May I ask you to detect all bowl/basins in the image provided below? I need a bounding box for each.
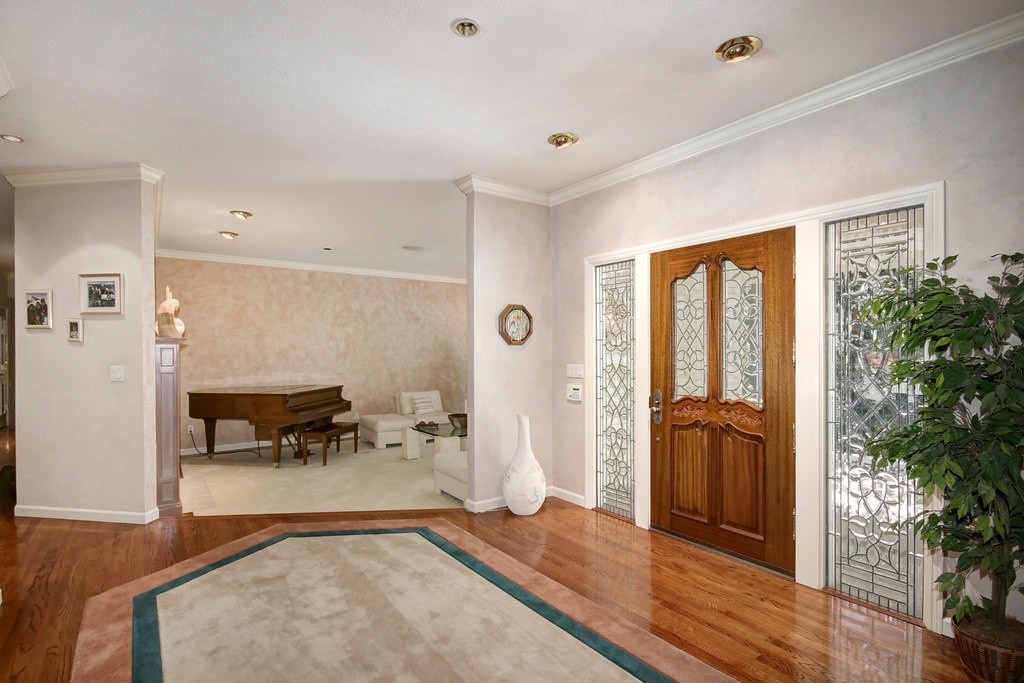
[448,413,467,429]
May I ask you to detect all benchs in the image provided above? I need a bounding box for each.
[302,420,359,466]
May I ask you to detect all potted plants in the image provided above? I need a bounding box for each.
[860,252,1022,683]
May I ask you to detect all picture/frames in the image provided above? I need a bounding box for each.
[79,272,125,315]
[68,319,85,343]
[23,289,54,330]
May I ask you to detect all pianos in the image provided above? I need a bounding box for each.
[185,383,352,468]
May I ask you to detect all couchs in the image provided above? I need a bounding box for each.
[361,389,458,450]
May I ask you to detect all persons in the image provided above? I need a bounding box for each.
[69,322,78,339]
[28,298,48,325]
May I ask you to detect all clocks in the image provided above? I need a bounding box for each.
[497,303,534,345]
